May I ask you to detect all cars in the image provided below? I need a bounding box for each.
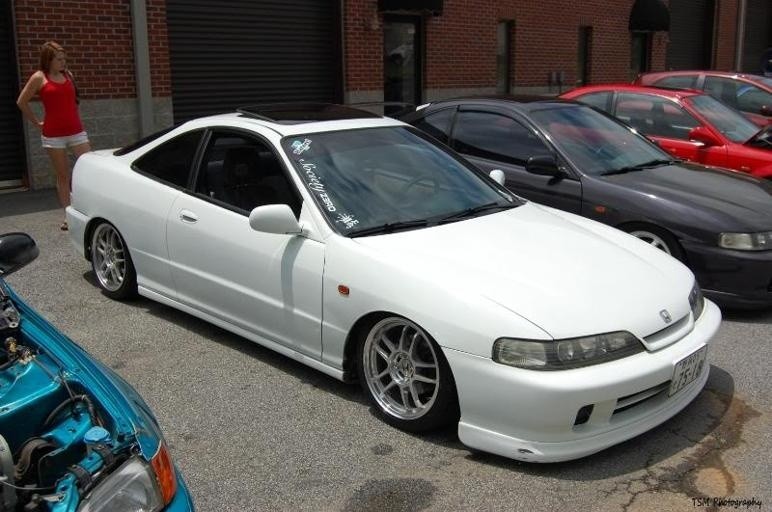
[2,227,197,510]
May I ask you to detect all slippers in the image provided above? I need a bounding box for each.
[61,221,67,229]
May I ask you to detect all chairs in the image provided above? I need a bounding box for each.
[630,103,688,140]
[216,144,282,212]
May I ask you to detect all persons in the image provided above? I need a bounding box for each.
[16,41,92,230]
